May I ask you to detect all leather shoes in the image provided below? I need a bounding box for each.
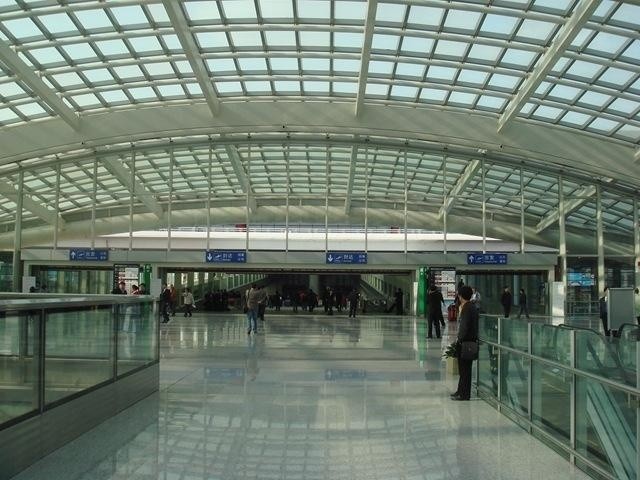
[450,392,470,400]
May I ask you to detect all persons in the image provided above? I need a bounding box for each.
[450,284,479,401]
[438,292,445,329]
[599,286,609,336]
[29,287,38,321]
[634,287,640,325]
[425,286,441,339]
[110,282,361,335]
[501,287,512,318]
[516,289,529,319]
[469,287,482,311]
[394,288,403,315]
[40,285,49,321]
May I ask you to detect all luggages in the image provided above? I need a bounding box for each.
[448,303,457,321]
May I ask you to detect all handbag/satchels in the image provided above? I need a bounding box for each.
[243,305,247,313]
[460,341,479,360]
